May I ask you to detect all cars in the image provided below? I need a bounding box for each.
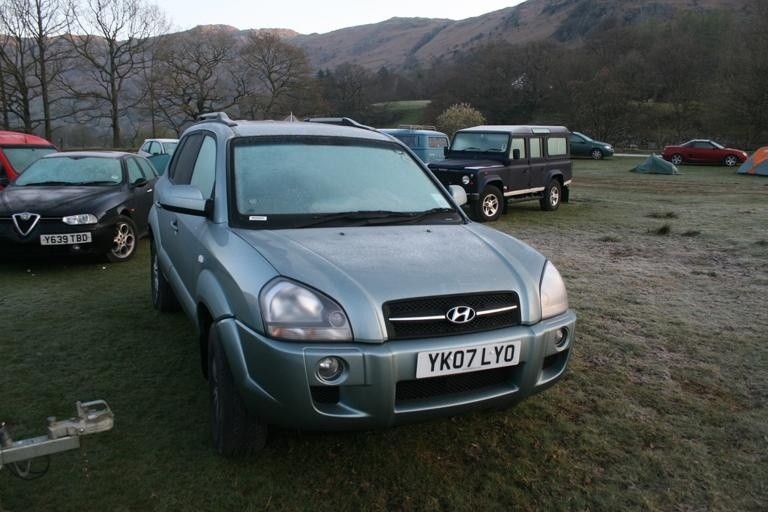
[0,129,181,261]
[661,140,748,167]
[570,132,614,160]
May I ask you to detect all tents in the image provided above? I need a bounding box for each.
[629,153,678,175]
[735,146,768,177]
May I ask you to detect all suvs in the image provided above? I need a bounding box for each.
[149,111,577,459]
[424,126,572,221]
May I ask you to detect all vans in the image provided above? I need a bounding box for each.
[377,128,450,164]
